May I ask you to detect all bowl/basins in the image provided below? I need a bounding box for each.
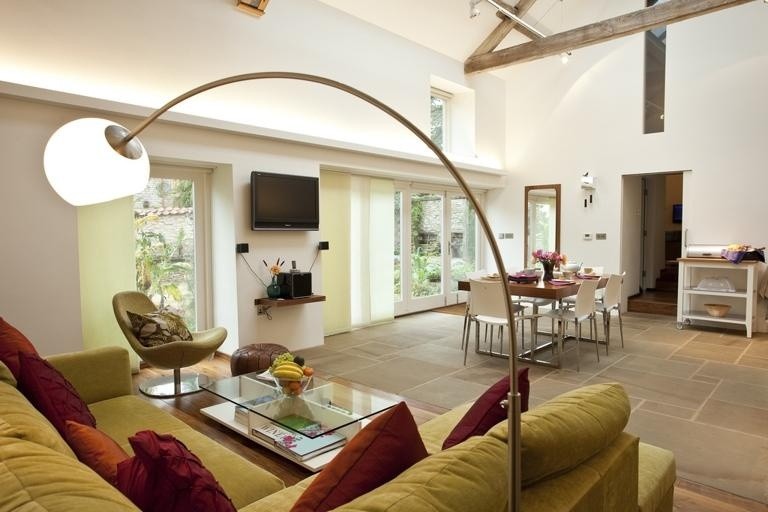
[703,304,731,317]
[274,376,311,398]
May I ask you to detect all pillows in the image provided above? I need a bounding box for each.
[0,318,40,382]
[287,402,426,512]
[115,428,237,512]
[125,304,195,348]
[438,366,529,450]
[15,348,95,434]
[67,418,130,486]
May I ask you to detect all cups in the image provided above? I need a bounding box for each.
[552,272,560,278]
[563,271,571,280]
[536,270,543,277]
[584,268,592,274]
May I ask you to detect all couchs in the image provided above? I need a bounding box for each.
[0,345,285,511]
[234,381,676,512]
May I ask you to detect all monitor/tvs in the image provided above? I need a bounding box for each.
[277,272,313,299]
[251,171,320,232]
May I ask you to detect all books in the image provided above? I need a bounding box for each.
[252,414,317,445]
[256,366,274,381]
[275,424,346,462]
[234,395,275,426]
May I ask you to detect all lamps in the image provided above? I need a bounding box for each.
[42,72,522,511]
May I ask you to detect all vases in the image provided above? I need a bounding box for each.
[542,263,554,281]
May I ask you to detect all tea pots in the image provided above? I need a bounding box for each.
[560,256,584,272]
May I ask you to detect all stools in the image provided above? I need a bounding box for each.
[230,342,291,375]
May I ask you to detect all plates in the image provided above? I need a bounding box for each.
[551,280,574,282]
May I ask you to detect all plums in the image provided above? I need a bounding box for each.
[294,356,304,366]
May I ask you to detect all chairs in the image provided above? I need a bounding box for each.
[461,266,626,373]
[110,291,226,398]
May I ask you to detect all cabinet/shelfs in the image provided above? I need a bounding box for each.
[676,258,758,338]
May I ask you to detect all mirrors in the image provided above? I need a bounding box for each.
[525,184,561,268]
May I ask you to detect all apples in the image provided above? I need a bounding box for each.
[292,387,302,395]
[303,368,313,376]
[288,382,299,390]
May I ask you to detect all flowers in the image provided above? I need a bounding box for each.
[531,249,566,269]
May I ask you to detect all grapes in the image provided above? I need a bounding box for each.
[269,352,294,373]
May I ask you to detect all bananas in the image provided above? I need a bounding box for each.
[272,361,304,379]
